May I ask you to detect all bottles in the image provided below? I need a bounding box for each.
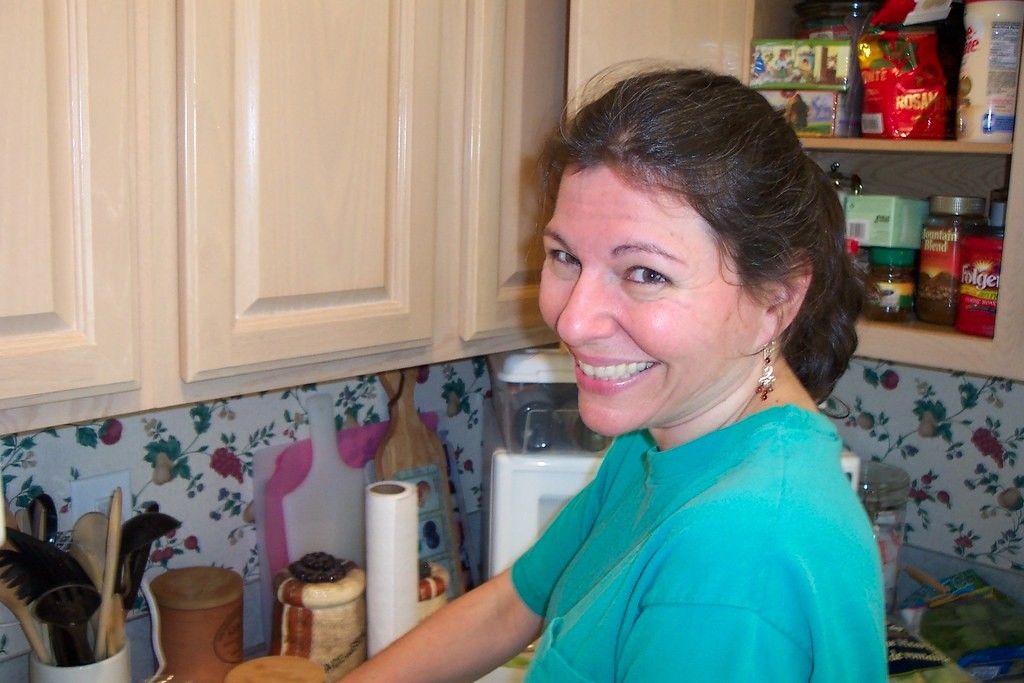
[857,461,910,618]
[955,1,1024,145]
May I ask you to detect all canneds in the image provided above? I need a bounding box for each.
[859,195,1008,339]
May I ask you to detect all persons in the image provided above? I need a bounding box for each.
[339,71,890,683]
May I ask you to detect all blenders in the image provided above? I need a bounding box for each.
[482,341,612,587]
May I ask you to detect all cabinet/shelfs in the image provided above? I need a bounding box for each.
[0,0,1024,435]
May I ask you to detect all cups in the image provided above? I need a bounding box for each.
[30,633,132,683]
[150,565,245,683]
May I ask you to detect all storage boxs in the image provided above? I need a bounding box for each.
[488,343,612,456]
[756,89,858,137]
[845,195,930,248]
[749,40,857,93]
